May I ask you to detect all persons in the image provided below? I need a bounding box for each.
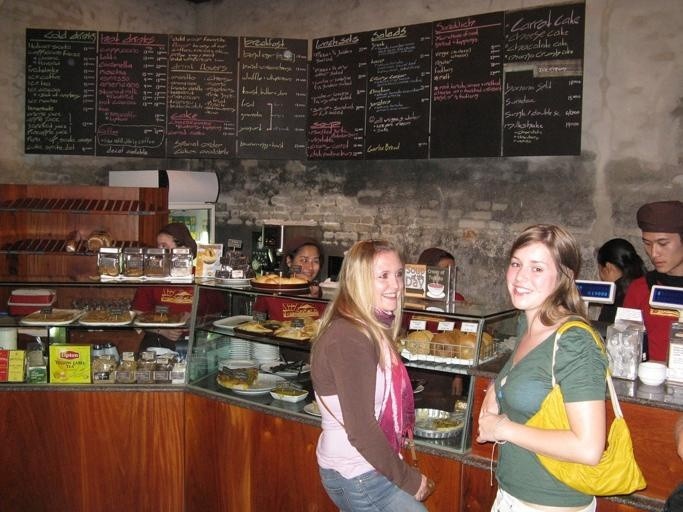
[666,419,682,512]
[475,221,612,512]
[596,237,649,331]
[308,240,437,511]
[621,200,682,362]
[255,236,330,334]
[131,221,198,362]
[401,247,467,398]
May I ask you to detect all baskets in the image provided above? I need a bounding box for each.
[397,339,499,369]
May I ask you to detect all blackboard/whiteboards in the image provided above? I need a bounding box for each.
[307,2,586,160]
[24,27,307,159]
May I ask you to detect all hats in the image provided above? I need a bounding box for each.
[417,247,446,269]
[284,235,324,269]
[162,219,197,261]
[636,201,682,232]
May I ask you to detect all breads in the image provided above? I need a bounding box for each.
[253,272,308,284]
[235,315,314,341]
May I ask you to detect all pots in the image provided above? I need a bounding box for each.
[87,229,110,251]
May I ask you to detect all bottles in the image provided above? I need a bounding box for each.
[200,221,208,241]
[91,342,185,384]
[664,321,683,386]
[168,214,196,239]
[202,257,216,282]
[97,247,190,278]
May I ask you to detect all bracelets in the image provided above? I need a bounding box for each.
[491,418,508,445]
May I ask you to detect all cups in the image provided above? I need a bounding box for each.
[635,384,673,402]
[74,296,135,310]
[638,361,674,385]
[427,283,444,295]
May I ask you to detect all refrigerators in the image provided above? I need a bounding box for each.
[107,169,220,244]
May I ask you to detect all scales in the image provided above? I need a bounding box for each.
[573,279,616,341]
[649,284,683,337]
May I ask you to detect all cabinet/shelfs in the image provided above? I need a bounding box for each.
[0,278,196,384]
[188,289,512,456]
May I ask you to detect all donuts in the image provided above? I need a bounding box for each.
[406,328,493,359]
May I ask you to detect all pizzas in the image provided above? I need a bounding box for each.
[216,366,259,390]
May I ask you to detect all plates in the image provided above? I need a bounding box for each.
[260,360,312,376]
[213,371,286,398]
[302,403,321,420]
[409,405,461,439]
[211,277,251,286]
[413,384,424,394]
[426,291,445,298]
[132,314,190,326]
[19,308,83,326]
[217,337,281,371]
[211,314,252,330]
[79,311,135,326]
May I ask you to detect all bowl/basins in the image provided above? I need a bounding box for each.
[269,387,308,403]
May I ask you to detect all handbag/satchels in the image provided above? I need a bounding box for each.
[523,383,646,497]
